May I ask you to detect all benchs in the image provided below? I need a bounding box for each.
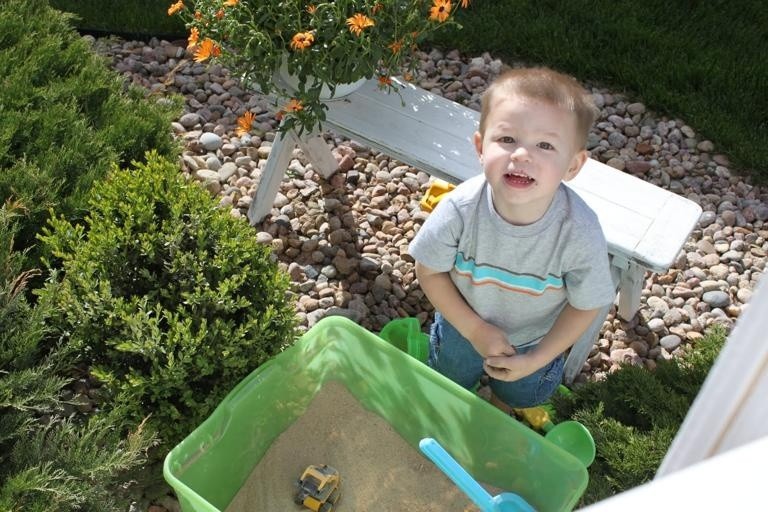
[241,70,703,383]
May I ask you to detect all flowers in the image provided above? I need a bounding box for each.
[168,0,470,138]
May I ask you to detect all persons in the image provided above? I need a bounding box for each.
[408,65,616,406]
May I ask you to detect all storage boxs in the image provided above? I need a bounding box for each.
[163,316,590,511]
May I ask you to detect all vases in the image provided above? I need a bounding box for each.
[278,64,367,100]
